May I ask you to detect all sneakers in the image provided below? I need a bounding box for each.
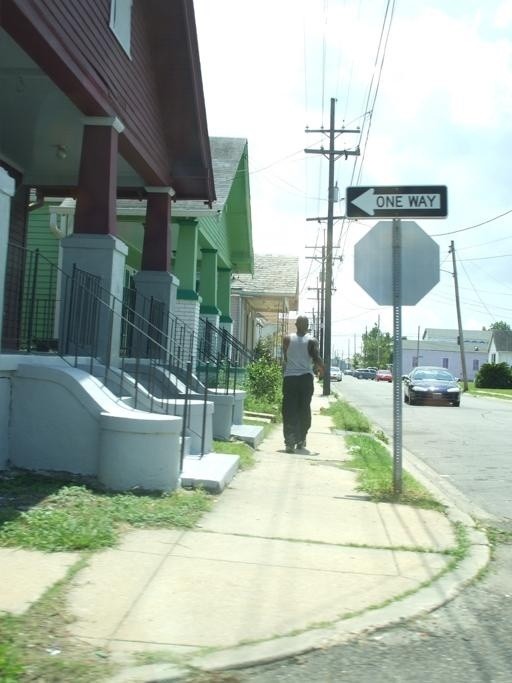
[286,443,295,452]
[297,440,306,448]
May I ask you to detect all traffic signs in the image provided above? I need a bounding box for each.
[346,185,448,219]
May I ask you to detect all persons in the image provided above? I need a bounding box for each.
[279,314,325,455]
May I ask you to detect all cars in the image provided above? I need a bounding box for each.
[344,367,393,382]
[319,366,342,381]
[404,365,461,407]
